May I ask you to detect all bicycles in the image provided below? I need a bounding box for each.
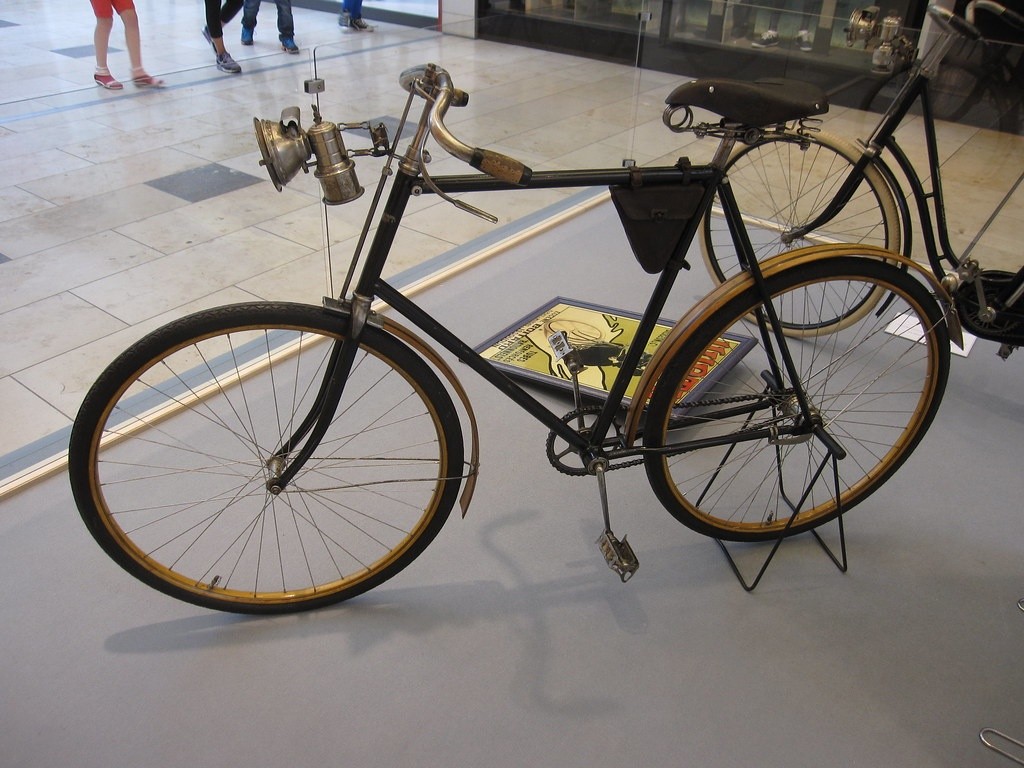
[698,1,1023,362]
[66,49,966,616]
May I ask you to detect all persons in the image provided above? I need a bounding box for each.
[202,0,244,74]
[90,0,166,90]
[340,0,377,35]
[242,1,303,56]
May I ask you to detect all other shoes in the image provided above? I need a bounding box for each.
[795,35,813,52]
[281,38,299,53]
[751,30,780,48]
[240,28,254,45]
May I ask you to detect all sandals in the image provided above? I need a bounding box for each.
[133,76,168,88]
[93,74,123,89]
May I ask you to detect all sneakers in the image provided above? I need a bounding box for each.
[216,52,242,73]
[201,26,219,56]
[338,11,374,33]
